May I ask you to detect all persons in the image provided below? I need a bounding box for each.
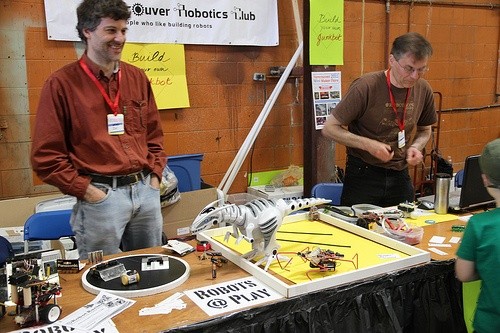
[455,139,500,333]
[322,32,437,208]
[30,0,167,258]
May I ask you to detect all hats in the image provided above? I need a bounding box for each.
[476,138,500,187]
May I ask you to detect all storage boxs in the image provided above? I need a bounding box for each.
[161,153,218,240]
[247,185,303,205]
[0,226,51,250]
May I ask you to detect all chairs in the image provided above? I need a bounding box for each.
[24,210,76,253]
[455,169,464,190]
[312,183,344,206]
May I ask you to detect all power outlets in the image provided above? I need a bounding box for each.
[253,72,266,81]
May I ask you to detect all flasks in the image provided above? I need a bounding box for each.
[434,173,451,214]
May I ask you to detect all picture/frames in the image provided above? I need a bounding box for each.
[197,211,431,298]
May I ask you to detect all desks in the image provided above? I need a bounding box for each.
[0,187,500,333]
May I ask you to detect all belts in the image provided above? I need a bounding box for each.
[79,167,151,186]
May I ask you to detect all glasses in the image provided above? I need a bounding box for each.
[390,53,430,75]
